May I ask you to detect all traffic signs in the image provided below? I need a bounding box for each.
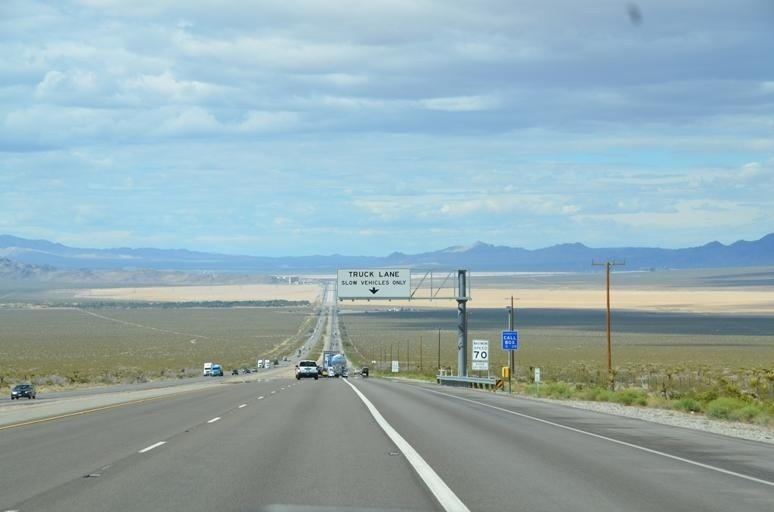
[336,268,412,298]
[501,330,518,350]
[472,340,488,361]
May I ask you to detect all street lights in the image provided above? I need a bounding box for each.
[504,305,513,371]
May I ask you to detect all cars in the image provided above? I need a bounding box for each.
[9,385,34,399]
[201,350,368,381]
